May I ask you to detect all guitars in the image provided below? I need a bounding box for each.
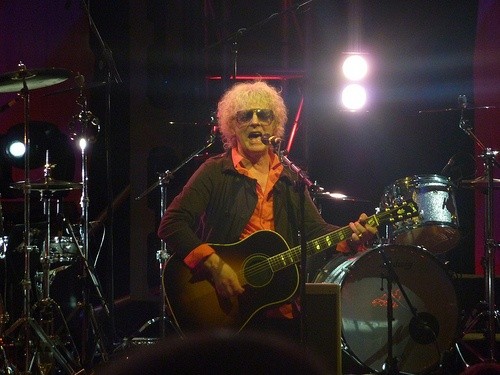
[162,198,419,348]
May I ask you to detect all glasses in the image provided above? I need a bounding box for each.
[236,109,275,125]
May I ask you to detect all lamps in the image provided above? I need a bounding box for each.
[0,121,44,170]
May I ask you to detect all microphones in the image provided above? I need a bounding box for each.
[441,155,455,174]
[458,95,467,111]
[261,134,282,145]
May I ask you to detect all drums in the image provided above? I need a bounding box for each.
[41,236,81,266]
[383,175,460,255]
[314,244,462,374]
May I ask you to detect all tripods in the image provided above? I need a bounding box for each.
[453,106,499,375]
[0,0,221,375]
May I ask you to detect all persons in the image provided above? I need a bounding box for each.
[157,81,380,337]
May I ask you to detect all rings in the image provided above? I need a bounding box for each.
[359,233,363,237]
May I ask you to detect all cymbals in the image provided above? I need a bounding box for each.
[9,181,81,190]
[417,105,496,114]
[458,179,500,189]
[0,68,70,92]
